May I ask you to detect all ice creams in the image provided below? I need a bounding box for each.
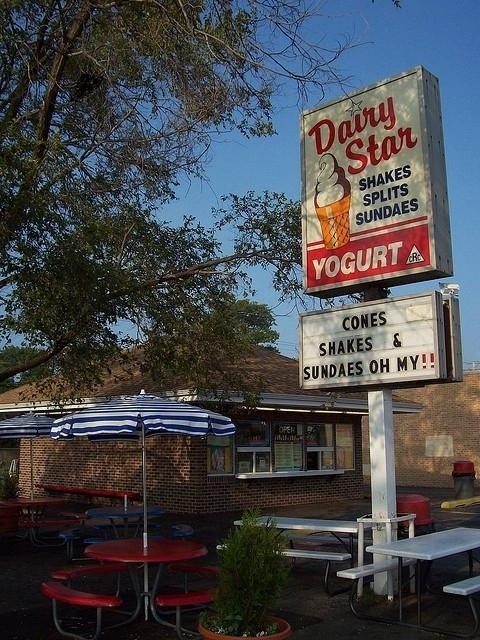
[315,153,352,250]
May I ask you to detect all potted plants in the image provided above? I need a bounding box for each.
[198,507,293,639]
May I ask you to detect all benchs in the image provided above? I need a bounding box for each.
[0,485,164,563]
[40,537,234,640]
[442,575,480,640]
[337,558,418,622]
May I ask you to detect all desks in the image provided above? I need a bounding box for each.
[365,526,479,627]
[215,515,371,596]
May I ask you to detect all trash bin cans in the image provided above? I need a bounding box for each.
[452,461,476,500]
[396,494,436,593]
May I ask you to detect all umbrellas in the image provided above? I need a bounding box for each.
[0,410,59,500]
[50,388,238,621]
